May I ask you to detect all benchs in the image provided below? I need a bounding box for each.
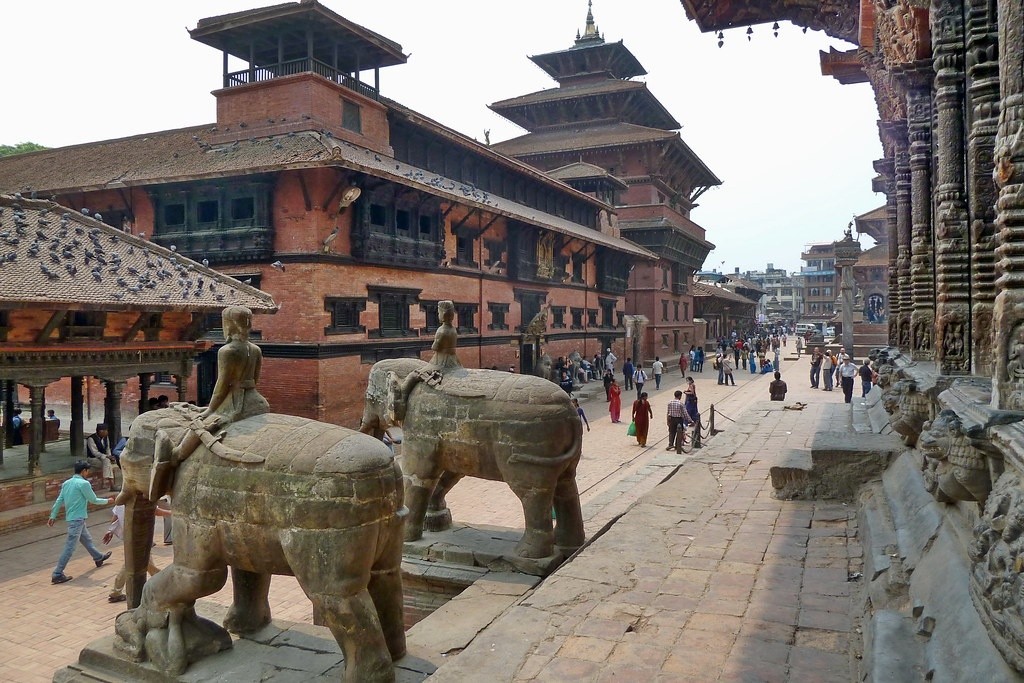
[0,463,116,503]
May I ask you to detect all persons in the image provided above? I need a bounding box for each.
[47,459,114,583]
[677,353,688,378]
[149,394,169,409]
[810,344,878,403]
[769,370,787,401]
[151,494,172,547]
[556,348,617,397]
[632,392,653,447]
[13,407,27,446]
[652,356,664,391]
[690,344,705,373]
[101,500,172,604]
[87,423,122,492]
[48,409,57,419]
[665,376,699,450]
[113,426,131,471]
[572,397,590,432]
[401,299,463,405]
[717,321,809,386]
[867,297,886,325]
[622,357,648,399]
[170,306,270,463]
[603,358,621,423]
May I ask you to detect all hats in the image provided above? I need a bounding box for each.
[843,357,850,359]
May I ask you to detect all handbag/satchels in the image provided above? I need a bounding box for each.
[627,418,636,435]
[716,366,718,370]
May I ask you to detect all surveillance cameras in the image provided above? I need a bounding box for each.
[272,261,281,267]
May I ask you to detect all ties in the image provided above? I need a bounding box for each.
[638,372,639,380]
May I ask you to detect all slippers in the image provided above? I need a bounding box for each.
[164,541,173,545]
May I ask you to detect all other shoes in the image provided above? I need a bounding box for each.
[95,550,112,567]
[52,576,73,583]
[811,385,814,388]
[814,386,818,389]
[110,486,120,492]
[666,446,674,450]
[718,382,724,385]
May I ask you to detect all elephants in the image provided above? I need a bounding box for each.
[114,404,406,683]
[358,357,587,557]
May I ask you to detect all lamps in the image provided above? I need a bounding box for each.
[339,186,361,208]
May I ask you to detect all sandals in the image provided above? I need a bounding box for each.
[108,594,127,602]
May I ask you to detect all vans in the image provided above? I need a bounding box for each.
[795,323,817,336]
[826,326,835,336]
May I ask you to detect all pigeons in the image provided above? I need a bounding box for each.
[171,112,501,211]
[0,191,284,301]
